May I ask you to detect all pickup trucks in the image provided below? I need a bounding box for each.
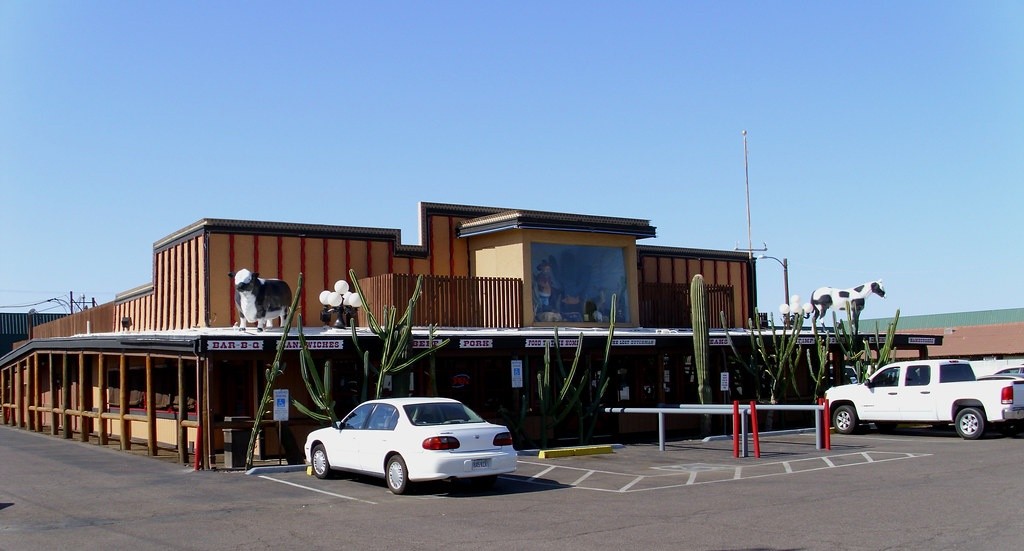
[820,359,1024,440]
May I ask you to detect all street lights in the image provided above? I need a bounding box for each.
[758,254,791,329]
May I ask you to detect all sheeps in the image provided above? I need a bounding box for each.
[227,267,292,333]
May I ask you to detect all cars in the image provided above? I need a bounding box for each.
[993,366,1024,377]
[304,397,518,495]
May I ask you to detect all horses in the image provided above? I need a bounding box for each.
[809,279,887,333]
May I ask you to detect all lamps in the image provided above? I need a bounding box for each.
[320,280,362,331]
[780,295,814,330]
